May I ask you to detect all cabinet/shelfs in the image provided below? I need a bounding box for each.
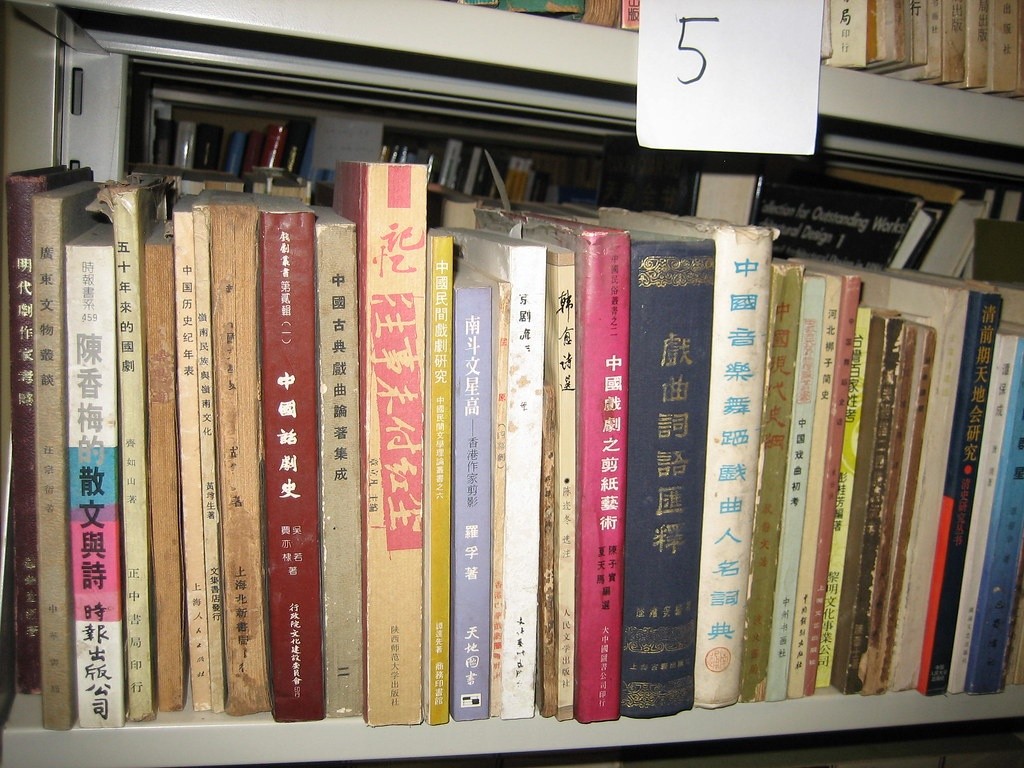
[0,0,1024,768]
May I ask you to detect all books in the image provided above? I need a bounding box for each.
[4,91,1022,726]
[451,0,1024,94]
